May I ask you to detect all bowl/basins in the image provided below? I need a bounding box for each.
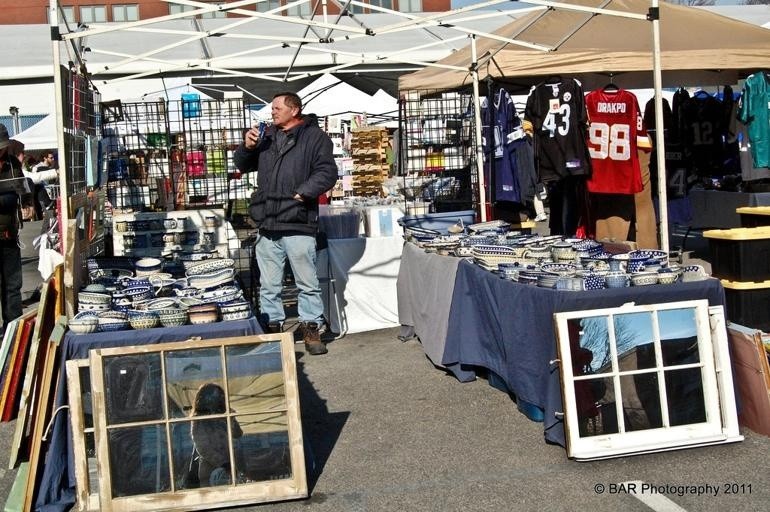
[82,259,238,302]
[67,291,253,336]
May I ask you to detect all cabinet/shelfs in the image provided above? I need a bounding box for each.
[112,209,230,262]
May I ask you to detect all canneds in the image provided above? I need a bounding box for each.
[258,122,267,140]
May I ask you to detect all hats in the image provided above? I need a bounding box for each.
[0,123,11,149]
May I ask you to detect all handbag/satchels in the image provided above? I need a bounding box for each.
[175,445,200,488]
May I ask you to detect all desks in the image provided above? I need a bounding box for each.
[318,200,430,237]
[34,252,266,511]
[397,232,726,445]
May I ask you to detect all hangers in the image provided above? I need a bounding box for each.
[477,58,770,105]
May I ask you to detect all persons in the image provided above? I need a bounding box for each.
[0,122,59,336]
[235,91,339,355]
[75,185,104,240]
[190,382,243,487]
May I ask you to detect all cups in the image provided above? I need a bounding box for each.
[116,216,216,257]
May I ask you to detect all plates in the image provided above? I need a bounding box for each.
[407,219,705,291]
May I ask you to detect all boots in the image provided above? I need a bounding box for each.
[267,321,284,333]
[301,321,327,355]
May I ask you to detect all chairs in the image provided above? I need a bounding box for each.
[241,231,331,328]
